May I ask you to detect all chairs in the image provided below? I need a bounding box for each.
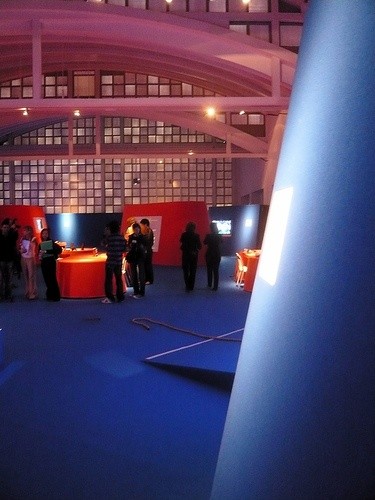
[235,253,248,285]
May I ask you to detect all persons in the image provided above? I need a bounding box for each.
[204,222,224,291]
[31,228,62,302]
[0,218,18,303]
[139,218,155,285]
[178,222,202,293]
[100,221,127,304]
[125,223,149,300]
[16,225,39,301]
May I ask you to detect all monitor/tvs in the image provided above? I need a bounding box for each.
[213,220,232,237]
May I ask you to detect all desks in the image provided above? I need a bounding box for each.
[234,250,259,293]
[56,248,128,297]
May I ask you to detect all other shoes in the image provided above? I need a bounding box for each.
[145,281,153,285]
[102,298,115,303]
[129,294,135,297]
[29,293,37,299]
[133,294,145,299]
[117,295,125,302]
